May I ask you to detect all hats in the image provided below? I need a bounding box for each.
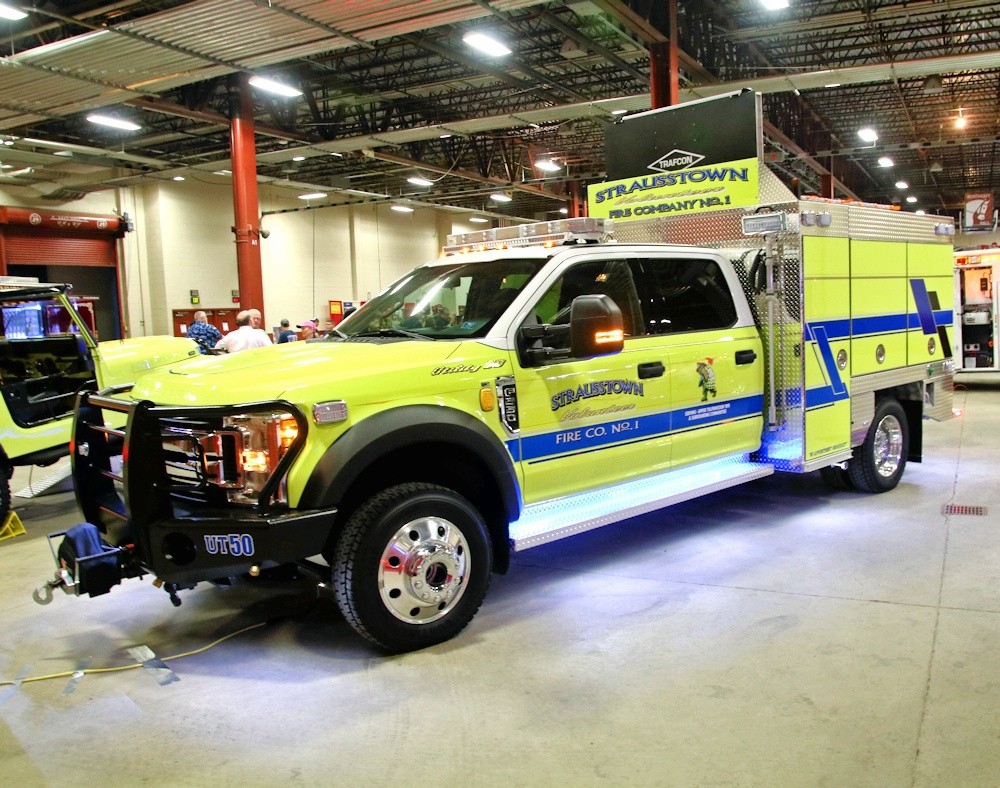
[296,321,316,333]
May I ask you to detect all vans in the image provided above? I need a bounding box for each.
[954,247,1000,389]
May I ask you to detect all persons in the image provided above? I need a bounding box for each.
[278,320,298,343]
[321,320,334,338]
[297,317,319,340]
[400,303,451,328]
[186,311,225,355]
[215,309,274,353]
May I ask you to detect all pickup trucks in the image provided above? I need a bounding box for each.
[29,86,962,657]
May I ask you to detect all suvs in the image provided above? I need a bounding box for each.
[0,275,229,531]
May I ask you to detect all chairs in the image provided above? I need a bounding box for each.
[637,273,673,335]
[484,288,545,351]
